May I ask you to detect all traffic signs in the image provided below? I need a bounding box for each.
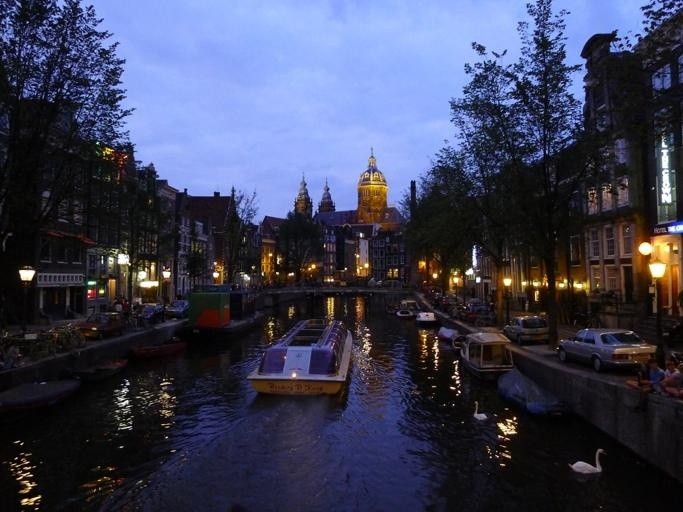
[638,242,667,371]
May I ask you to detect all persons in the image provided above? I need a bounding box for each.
[112,296,129,320]
[637,359,683,398]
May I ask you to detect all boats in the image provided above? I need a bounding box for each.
[383,294,437,327]
[136,310,264,357]
[246,319,353,395]
[439,327,566,413]
[0,359,128,413]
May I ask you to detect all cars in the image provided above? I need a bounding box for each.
[383,279,401,288]
[503,316,549,345]
[79,300,191,340]
[558,328,658,373]
[430,289,497,326]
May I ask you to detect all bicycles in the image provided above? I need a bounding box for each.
[0,320,86,369]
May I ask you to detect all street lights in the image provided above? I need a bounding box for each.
[252,266,256,289]
[162,271,171,306]
[18,266,36,332]
[503,278,511,326]
[453,276,458,303]
[364,263,370,278]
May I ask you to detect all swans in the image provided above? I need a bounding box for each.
[568,448,608,473]
[473,401,487,420]
